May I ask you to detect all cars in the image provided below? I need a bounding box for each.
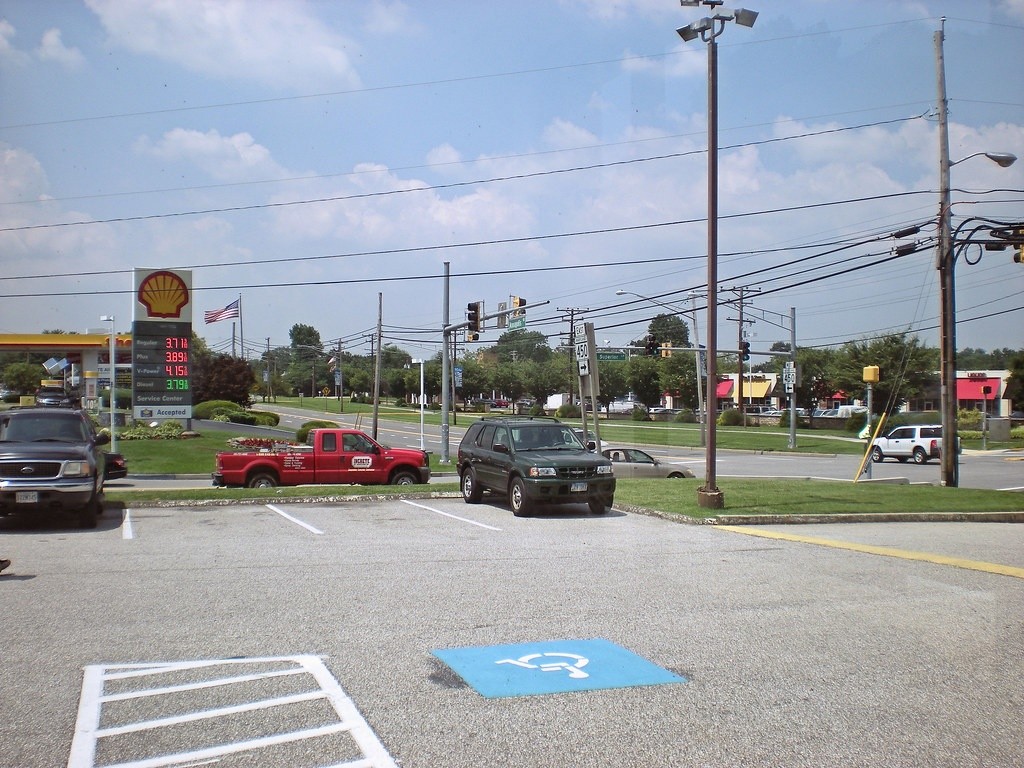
[100,447,127,479]
[467,393,868,418]
[32,386,72,408]
[561,427,609,453]
[0,391,22,401]
[594,446,696,479]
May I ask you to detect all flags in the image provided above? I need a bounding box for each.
[204,299,239,324]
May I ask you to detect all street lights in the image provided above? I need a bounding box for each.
[933,29,1018,488]
[615,288,706,447]
[687,291,799,449]
[100,315,115,454]
[676,0,760,509]
[412,358,425,451]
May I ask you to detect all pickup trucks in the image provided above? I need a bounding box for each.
[211,428,431,489]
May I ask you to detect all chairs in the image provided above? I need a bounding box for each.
[535,432,553,446]
[57,423,79,438]
[501,434,514,450]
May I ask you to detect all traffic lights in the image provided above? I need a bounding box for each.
[468,333,479,342]
[743,341,750,360]
[468,301,481,332]
[984,386,992,394]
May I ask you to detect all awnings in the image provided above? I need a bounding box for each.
[956,378,1000,400]
[731,379,771,397]
[715,380,733,396]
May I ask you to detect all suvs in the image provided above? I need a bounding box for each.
[871,424,962,464]
[456,413,617,516]
[0,407,109,530]
